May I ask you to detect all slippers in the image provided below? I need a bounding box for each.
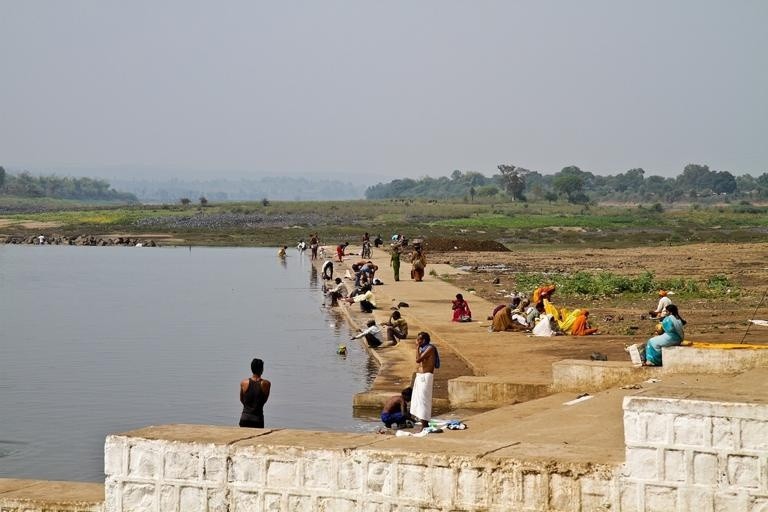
[642,361,657,367]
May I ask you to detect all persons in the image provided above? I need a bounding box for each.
[38,233,45,246]
[386,310,408,347]
[390,246,400,281]
[309,234,317,242]
[451,293,471,321]
[408,331,437,434]
[380,387,417,428]
[648,290,673,323]
[391,234,401,242]
[297,241,306,251]
[239,358,271,427]
[640,304,687,367]
[336,242,350,262]
[314,232,320,244]
[359,263,378,284]
[402,236,408,248]
[406,243,427,282]
[352,260,372,286]
[279,246,288,258]
[328,278,349,308]
[374,232,384,247]
[310,238,318,260]
[362,232,370,259]
[489,285,599,335]
[350,318,384,348]
[345,285,377,314]
[322,261,334,279]
